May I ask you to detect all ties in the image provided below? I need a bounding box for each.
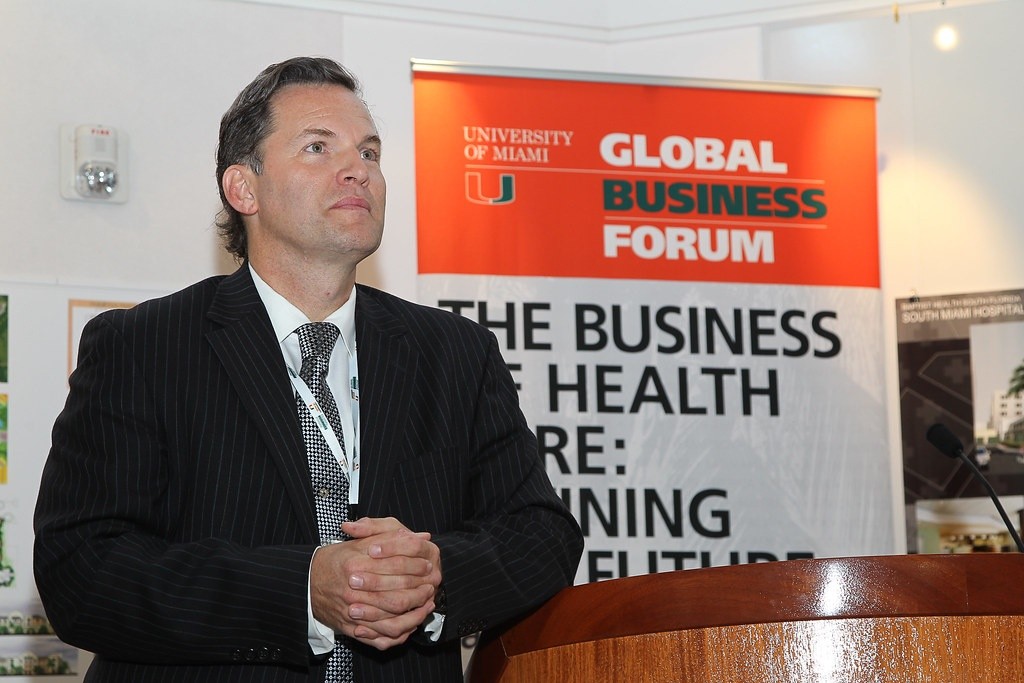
[298,325,352,683]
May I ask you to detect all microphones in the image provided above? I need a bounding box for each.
[927,423,1024,553]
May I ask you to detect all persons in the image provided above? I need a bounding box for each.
[32,56,585,683]
